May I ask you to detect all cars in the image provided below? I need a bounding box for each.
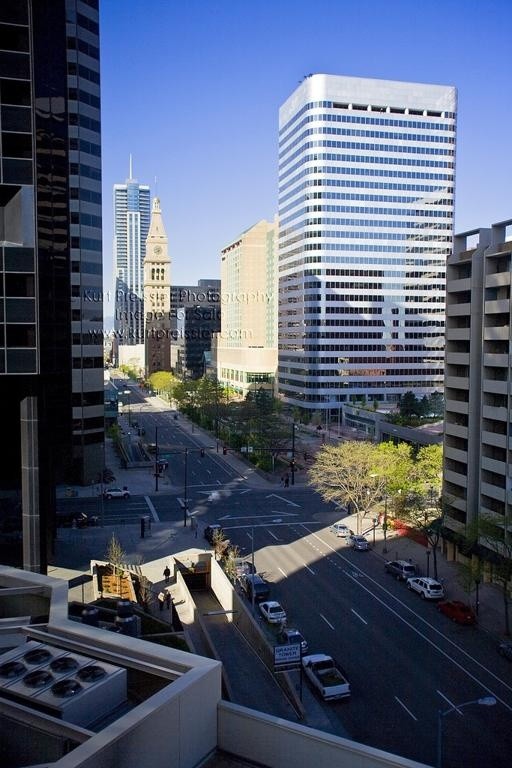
[225,559,250,578]
[278,628,309,655]
[384,560,418,581]
[346,534,369,551]
[204,525,233,554]
[258,601,287,624]
[436,600,476,625]
[330,523,351,537]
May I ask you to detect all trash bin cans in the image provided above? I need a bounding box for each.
[174,415,177,420]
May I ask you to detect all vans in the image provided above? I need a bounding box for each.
[240,574,269,601]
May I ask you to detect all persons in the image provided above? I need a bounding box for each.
[280,474,285,488]
[165,591,171,610]
[158,591,167,611]
[285,476,289,488]
[162,565,170,584]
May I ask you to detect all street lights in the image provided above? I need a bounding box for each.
[155,423,180,491]
[291,421,299,485]
[140,403,152,440]
[473,576,482,618]
[184,445,215,526]
[436,695,497,768]
[372,517,377,546]
[423,547,433,578]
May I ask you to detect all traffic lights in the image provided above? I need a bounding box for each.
[200,448,204,458]
[223,446,227,456]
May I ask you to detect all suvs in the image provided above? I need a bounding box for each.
[406,577,445,600]
[104,488,131,500]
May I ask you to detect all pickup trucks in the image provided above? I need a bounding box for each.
[301,654,351,701]
